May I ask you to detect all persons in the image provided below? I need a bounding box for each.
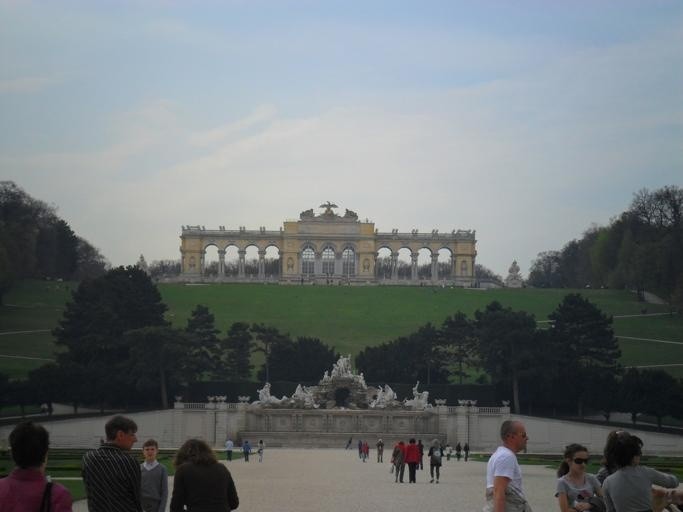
[226,438,233,461]
[242,440,251,461]
[0,422,72,512]
[554,430,679,512]
[80,413,143,511]
[258,440,264,462]
[486,421,529,512]
[140,439,167,512]
[170,439,239,511]
[344,436,470,483]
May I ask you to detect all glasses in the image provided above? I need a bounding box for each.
[575,459,588,465]
[508,431,526,437]
